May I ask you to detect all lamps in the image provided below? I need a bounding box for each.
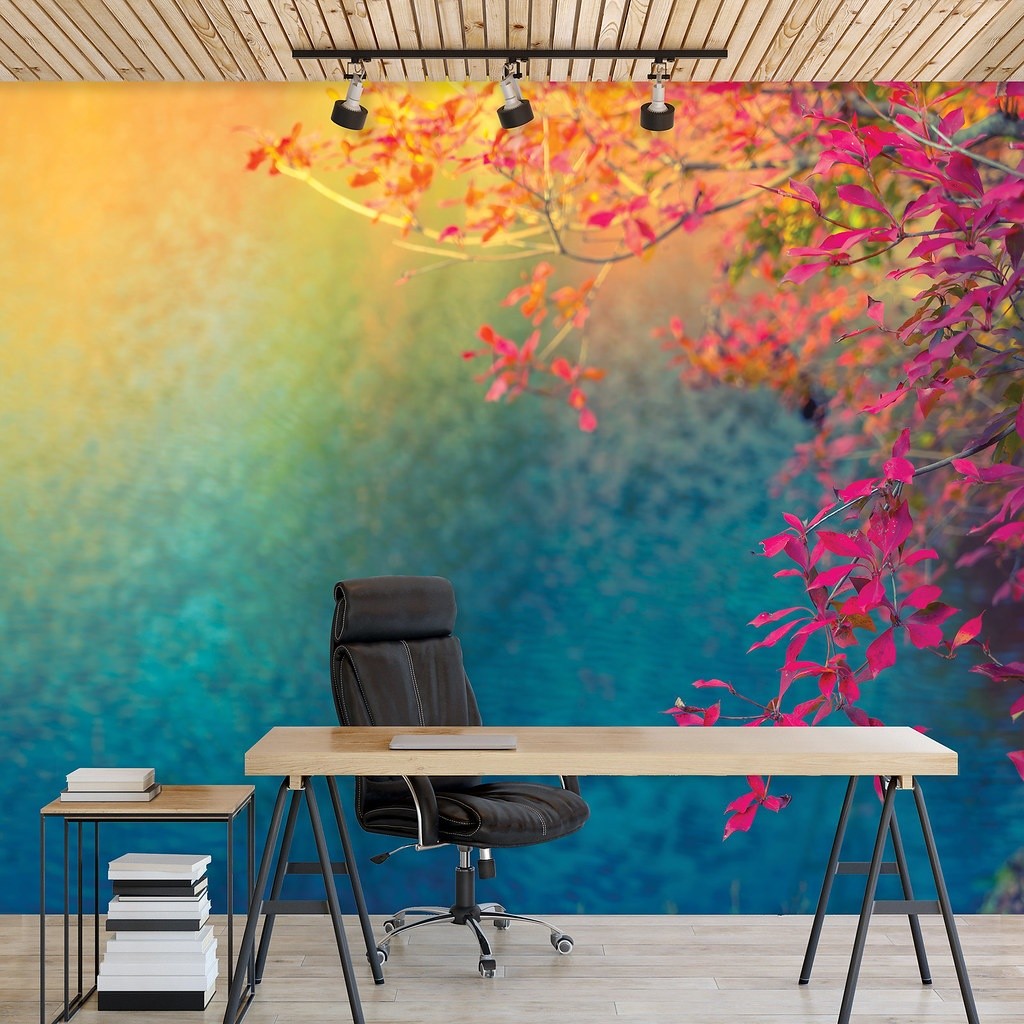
[497,58,534,129]
[640,59,674,132]
[331,58,368,130]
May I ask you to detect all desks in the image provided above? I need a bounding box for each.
[40,784,257,1024]
[223,726,979,1024]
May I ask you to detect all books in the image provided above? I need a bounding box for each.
[59,767,162,802]
[97,851,219,1012]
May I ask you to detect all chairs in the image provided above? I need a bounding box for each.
[331,574,589,982]
